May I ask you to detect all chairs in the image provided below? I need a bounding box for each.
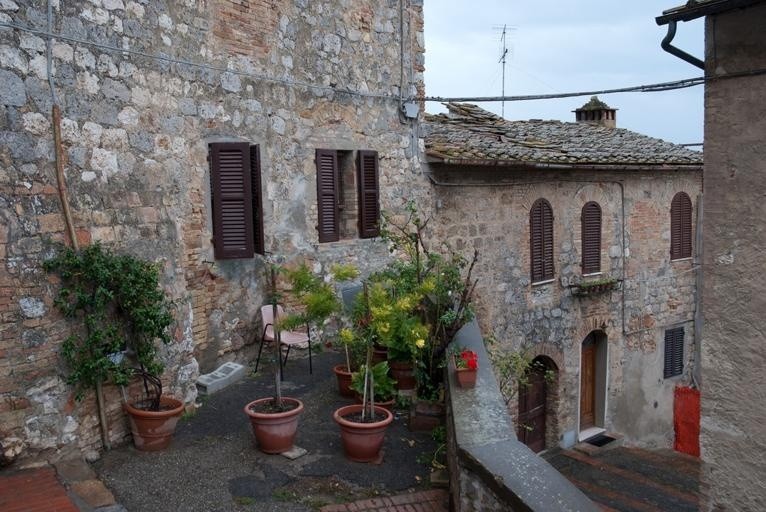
[255,304,314,374]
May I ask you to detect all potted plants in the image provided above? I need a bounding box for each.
[45,240,183,452]
[244,252,305,454]
